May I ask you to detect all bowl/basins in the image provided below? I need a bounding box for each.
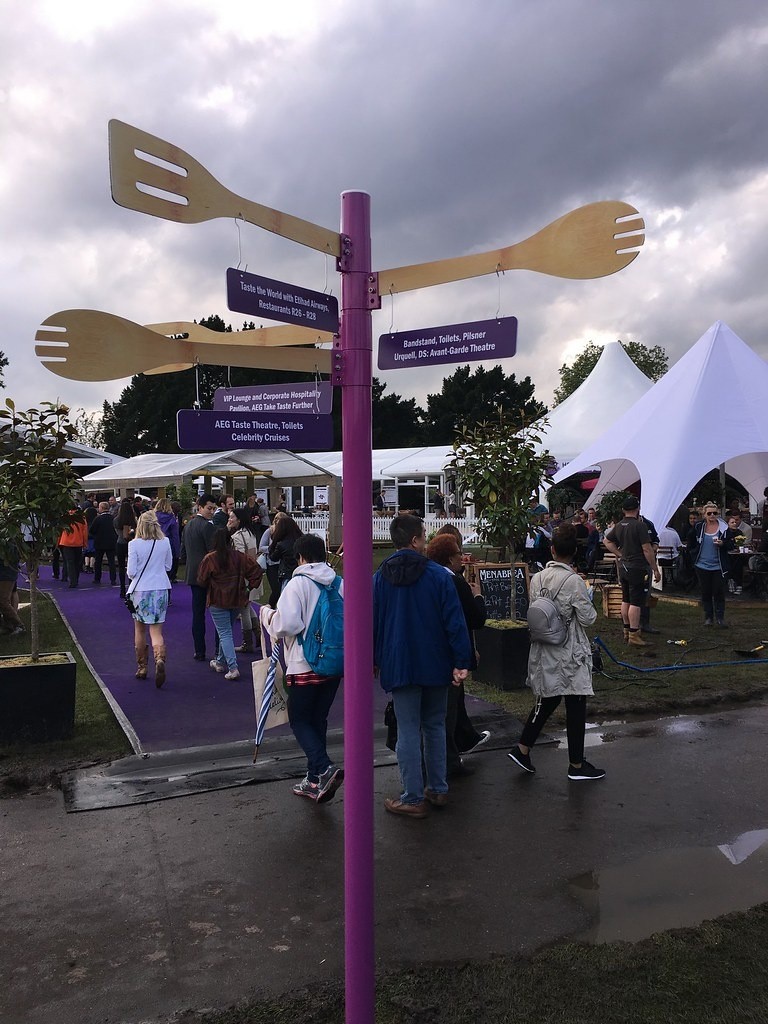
[749,550,753,554]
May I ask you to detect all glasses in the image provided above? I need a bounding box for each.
[705,511,718,516]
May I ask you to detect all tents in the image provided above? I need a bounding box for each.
[532,319,768,535]
[507,342,655,461]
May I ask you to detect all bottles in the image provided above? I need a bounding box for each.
[754,516,757,526]
[744,543,754,551]
[734,544,739,552]
[758,516,762,526]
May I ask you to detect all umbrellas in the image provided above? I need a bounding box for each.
[253,611,282,763]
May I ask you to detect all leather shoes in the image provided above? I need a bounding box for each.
[385,796,427,818]
[424,785,448,807]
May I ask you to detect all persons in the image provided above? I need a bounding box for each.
[90,497,137,598]
[153,498,181,606]
[197,527,263,679]
[658,487,768,629]
[259,513,288,607]
[51,492,100,588]
[184,494,271,661]
[375,490,386,510]
[127,510,172,687]
[268,517,304,596]
[133,496,159,521]
[273,493,287,512]
[603,497,664,645]
[526,500,603,536]
[433,489,456,518]
[262,533,344,804]
[0,520,27,634]
[372,514,606,818]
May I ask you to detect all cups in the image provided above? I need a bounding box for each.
[713,537,718,543]
[745,548,748,553]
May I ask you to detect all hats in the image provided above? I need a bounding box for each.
[623,496,639,510]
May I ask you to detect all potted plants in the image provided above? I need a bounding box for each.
[436,399,558,687]
[0,397,97,742]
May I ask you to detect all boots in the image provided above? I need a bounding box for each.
[234,629,253,653]
[135,644,149,679]
[119,572,127,598]
[642,605,661,634]
[251,618,262,648]
[152,644,167,688]
[624,626,656,646]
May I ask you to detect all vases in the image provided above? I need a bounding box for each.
[737,539,744,546]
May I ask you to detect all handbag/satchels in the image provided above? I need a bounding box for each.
[125,595,136,614]
[122,525,134,542]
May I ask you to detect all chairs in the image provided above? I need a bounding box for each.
[582,516,768,618]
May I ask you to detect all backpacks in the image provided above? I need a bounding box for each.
[294,574,346,677]
[527,573,574,645]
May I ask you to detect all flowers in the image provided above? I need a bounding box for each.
[735,536,747,541]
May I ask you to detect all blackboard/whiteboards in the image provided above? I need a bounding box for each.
[473,562,530,619]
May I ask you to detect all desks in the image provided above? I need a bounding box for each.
[727,550,765,590]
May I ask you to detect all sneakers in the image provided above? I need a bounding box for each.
[292,772,320,799]
[507,745,536,774]
[224,668,240,679]
[568,760,606,781]
[210,659,224,673]
[316,763,344,803]
[462,730,490,754]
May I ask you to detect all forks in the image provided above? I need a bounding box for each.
[378,200,645,296]
[34,309,333,382]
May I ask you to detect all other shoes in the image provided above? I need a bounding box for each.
[52,566,121,589]
[705,619,712,626]
[717,618,727,627]
[193,652,205,661]
[727,578,743,595]
